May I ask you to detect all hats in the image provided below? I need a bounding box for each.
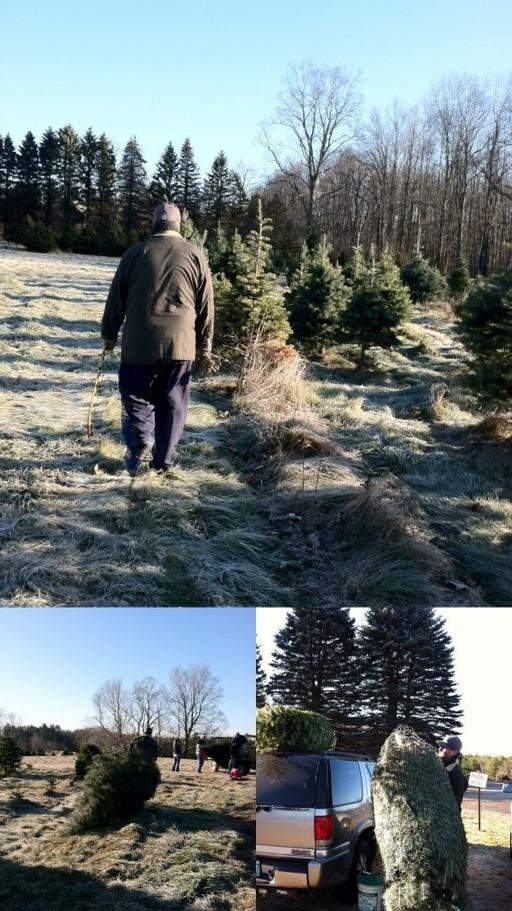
[153,205,181,231]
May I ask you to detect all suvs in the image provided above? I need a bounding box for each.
[257,749,377,906]
[205,735,257,771]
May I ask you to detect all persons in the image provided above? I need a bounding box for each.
[435,735,468,808]
[130,727,158,760]
[172,736,184,771]
[102,203,217,477]
[231,732,248,752]
[230,764,241,780]
[195,735,208,773]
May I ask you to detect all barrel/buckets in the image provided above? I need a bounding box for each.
[357,870,386,911]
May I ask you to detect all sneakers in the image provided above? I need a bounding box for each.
[128,441,151,475]
